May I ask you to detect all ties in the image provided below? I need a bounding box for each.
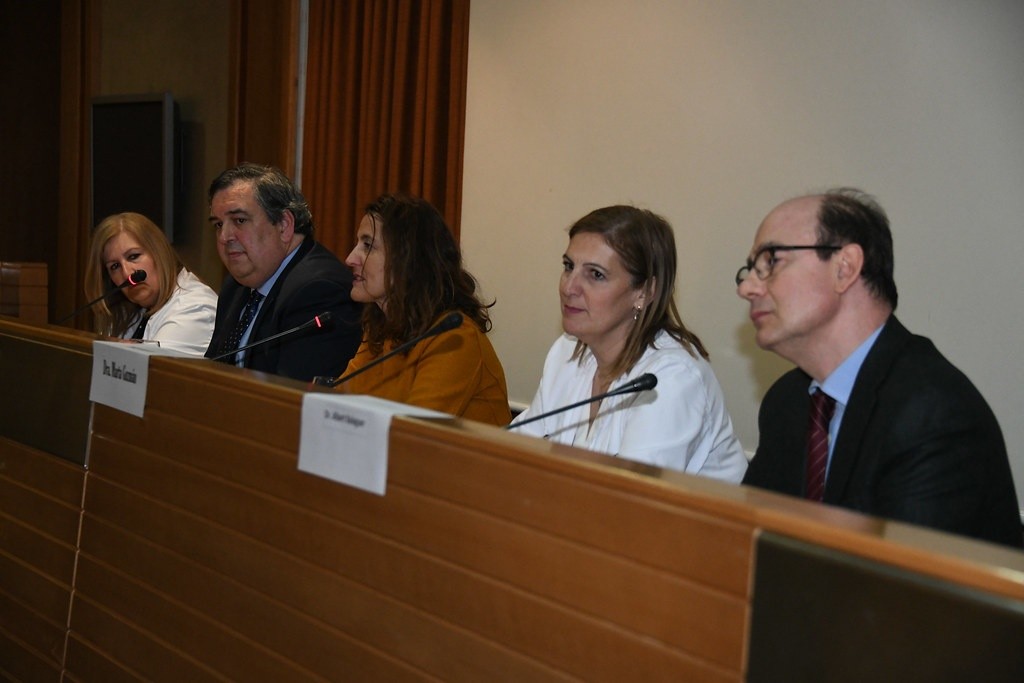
[807,387,838,503]
[217,289,263,364]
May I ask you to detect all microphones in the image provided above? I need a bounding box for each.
[506,373,658,429]
[211,312,335,361]
[328,313,463,388]
[54,270,147,325]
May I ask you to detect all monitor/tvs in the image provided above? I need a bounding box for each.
[89,92,174,243]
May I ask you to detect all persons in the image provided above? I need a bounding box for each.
[511,204,748,483]
[203,163,363,384]
[740,188,1024,551]
[332,194,511,428]
[83,212,218,358]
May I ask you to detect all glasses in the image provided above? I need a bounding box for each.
[736,245,841,287]
